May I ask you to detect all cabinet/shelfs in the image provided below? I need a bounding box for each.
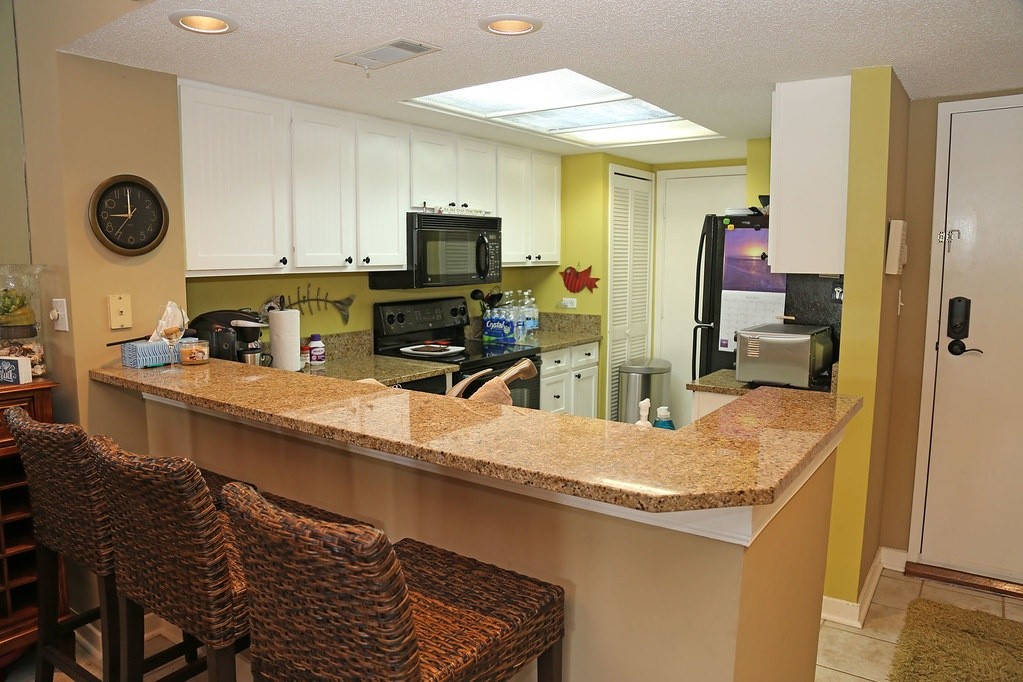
[178,78,562,277]
[607,163,655,423]
[540,342,599,418]
[768,75,851,275]
[0,376,77,682]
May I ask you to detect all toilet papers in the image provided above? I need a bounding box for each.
[268,308,301,371]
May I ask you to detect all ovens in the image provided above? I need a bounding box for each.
[733,323,833,388]
[398,358,539,411]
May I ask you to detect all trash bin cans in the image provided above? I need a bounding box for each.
[615,357,672,426]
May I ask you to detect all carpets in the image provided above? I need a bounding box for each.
[885,597,1023,682]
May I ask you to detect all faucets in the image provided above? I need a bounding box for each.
[443,356,539,404]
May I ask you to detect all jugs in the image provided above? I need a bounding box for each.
[236,347,273,367]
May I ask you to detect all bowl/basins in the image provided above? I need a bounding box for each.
[179,340,210,365]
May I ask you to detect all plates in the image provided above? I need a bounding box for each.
[400,345,465,357]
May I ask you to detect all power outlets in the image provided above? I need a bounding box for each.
[50,298,69,333]
[561,298,577,309]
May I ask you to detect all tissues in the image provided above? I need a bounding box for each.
[120,301,199,368]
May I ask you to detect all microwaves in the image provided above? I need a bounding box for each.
[368,211,503,290]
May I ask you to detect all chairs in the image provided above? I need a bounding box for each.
[4,405,198,682]
[220,481,566,682]
[88,432,374,682]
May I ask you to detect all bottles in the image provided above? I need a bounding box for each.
[308,334,326,366]
[483,341,532,358]
[300,346,311,363]
[482,289,539,343]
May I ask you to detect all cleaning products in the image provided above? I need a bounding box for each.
[634,398,675,430]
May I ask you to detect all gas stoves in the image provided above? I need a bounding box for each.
[373,296,541,372]
